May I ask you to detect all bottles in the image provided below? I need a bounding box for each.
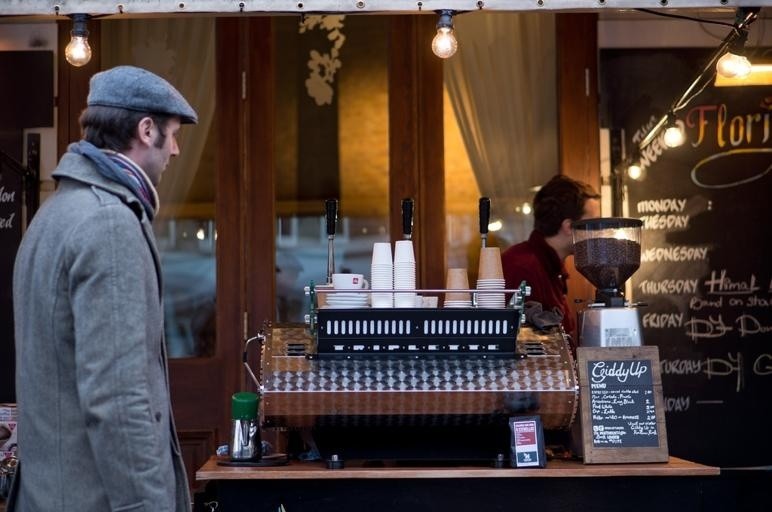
[260,414,290,464]
[304,286,318,325]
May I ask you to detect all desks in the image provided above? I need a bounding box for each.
[195,443,720,510]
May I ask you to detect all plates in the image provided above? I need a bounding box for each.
[321,292,369,309]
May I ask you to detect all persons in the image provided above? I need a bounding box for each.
[502,172,599,351]
[9,64,200,512]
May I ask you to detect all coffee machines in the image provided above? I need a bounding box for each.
[571,218,648,347]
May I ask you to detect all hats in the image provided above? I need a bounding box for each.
[85,62,201,127]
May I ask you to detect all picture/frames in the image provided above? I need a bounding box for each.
[576,346,669,464]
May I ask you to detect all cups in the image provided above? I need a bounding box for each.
[370,242,393,308]
[477,247,506,310]
[415,296,425,308]
[394,240,416,308]
[423,296,439,308]
[444,268,472,308]
[229,392,259,461]
[332,274,368,290]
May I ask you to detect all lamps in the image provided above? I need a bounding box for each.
[716,25,753,79]
[64,13,92,67]
[665,111,685,148]
[431,9,458,59]
[627,155,642,183]
